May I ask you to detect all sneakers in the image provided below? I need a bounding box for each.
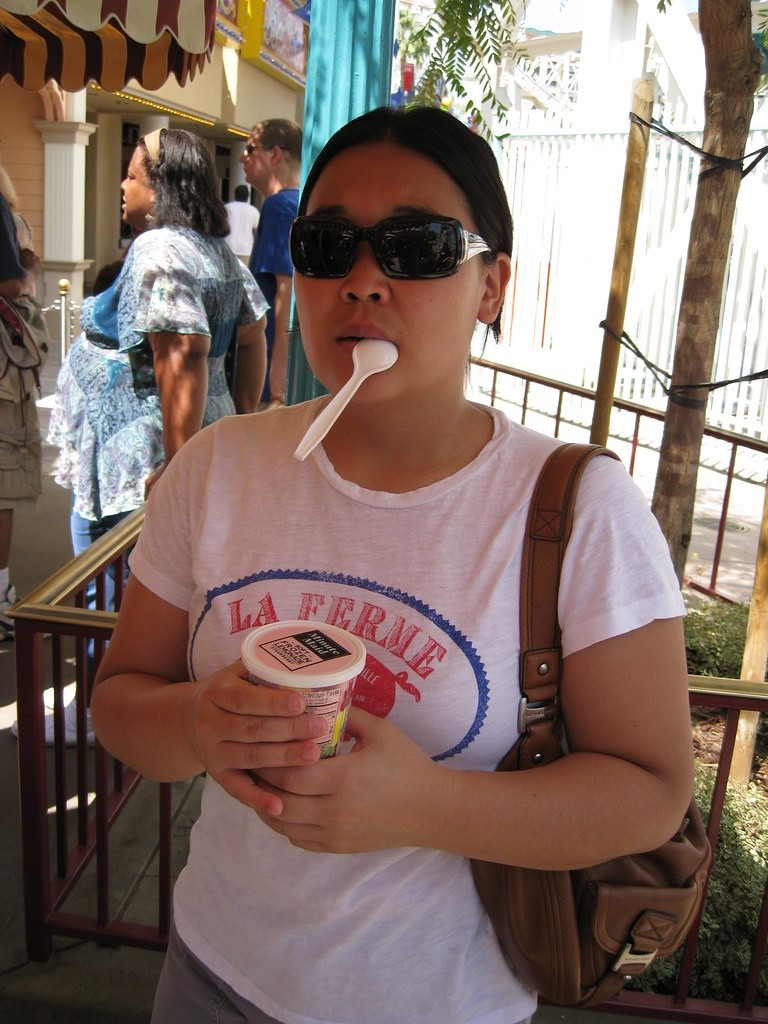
[0,584,52,640]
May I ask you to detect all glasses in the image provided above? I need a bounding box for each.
[289,214,492,280]
[246,144,291,156]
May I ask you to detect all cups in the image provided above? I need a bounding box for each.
[241,620,367,759]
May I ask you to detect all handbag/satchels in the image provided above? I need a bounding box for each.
[471,443,713,1008]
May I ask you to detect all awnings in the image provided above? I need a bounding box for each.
[0,0,216,94]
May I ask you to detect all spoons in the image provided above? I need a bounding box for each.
[293,339,399,462]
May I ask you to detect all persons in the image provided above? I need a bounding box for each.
[93,241,131,297]
[225,118,303,410]
[11,127,271,745]
[0,166,49,655]
[377,237,452,274]
[89,106,695,1024]
[224,184,261,267]
